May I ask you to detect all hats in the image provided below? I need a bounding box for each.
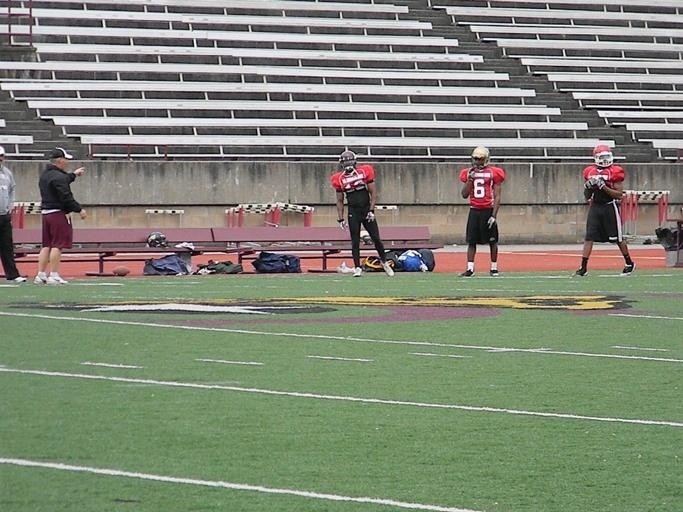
[0,146,6,155]
[48,147,74,159]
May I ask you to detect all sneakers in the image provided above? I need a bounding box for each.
[352,266,362,277]
[33,275,48,285]
[380,259,395,277]
[46,276,68,285]
[571,264,589,277]
[6,276,28,285]
[457,268,476,278]
[489,268,499,277]
[620,261,636,277]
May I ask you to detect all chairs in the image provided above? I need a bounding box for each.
[2,223,446,276]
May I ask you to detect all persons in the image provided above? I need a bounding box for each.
[330,151,395,278]
[33,148,87,285]
[458,146,505,278]
[572,145,637,277]
[0,146,28,283]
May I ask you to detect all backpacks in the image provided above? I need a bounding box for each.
[360,248,435,272]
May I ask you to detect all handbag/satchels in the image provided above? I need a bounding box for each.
[142,254,242,276]
[251,250,303,273]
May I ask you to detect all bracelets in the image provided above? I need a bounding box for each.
[368,210,375,213]
[337,219,346,222]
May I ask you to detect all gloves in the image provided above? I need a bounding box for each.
[365,210,375,223]
[336,217,348,232]
[583,177,597,190]
[467,168,475,182]
[596,177,606,190]
[487,215,496,229]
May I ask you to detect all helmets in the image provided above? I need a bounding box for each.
[471,146,490,170]
[339,150,357,174]
[592,144,614,168]
[147,231,168,248]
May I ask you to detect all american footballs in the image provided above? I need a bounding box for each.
[113,267,130,276]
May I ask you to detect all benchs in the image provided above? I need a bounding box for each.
[1,1,683,166]
